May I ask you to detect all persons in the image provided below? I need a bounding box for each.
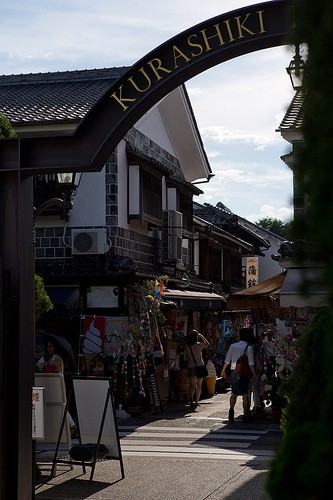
[250,328,277,413]
[36,341,78,438]
[221,328,257,424]
[183,329,211,409]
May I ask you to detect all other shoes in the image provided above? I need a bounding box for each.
[253,406,259,414]
[191,404,195,408]
[228,407,234,422]
[244,410,251,417]
[259,402,265,410]
[196,403,200,406]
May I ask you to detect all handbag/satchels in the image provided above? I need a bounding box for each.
[235,345,254,378]
[195,364,208,378]
[254,355,263,374]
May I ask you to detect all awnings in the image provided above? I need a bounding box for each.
[229,269,295,308]
[160,286,223,301]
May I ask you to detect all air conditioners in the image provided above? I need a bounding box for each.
[160,209,182,236]
[71,228,107,254]
[160,234,182,264]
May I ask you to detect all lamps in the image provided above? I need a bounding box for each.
[32,172,84,226]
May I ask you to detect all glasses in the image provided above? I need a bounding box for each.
[48,345,54,348]
